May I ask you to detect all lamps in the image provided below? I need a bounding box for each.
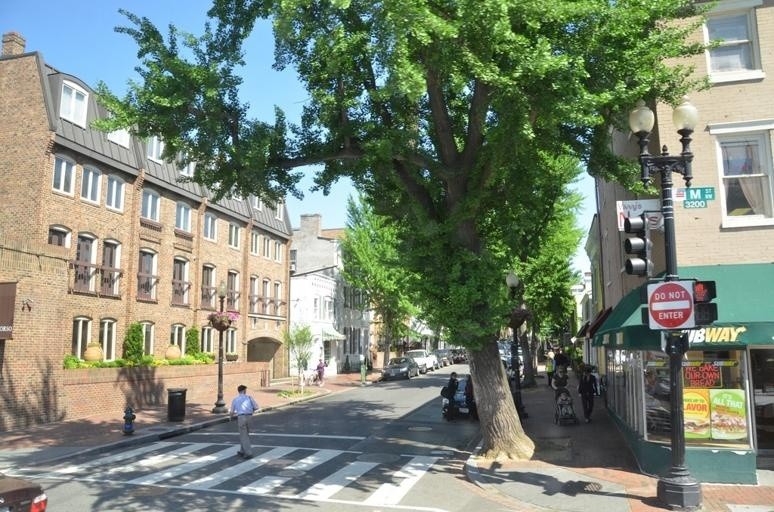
[22,298,34,305]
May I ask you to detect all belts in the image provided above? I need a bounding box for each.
[238,413,253,416]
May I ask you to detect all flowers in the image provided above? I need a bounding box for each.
[207,311,240,327]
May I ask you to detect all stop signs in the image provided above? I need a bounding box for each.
[647,280,695,330]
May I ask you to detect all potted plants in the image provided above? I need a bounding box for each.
[83,341,104,362]
[226,351,239,361]
[165,344,181,359]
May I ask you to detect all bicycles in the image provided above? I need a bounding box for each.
[308,368,325,387]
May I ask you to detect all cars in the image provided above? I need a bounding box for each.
[382,349,466,381]
[498,341,524,369]
[0,473,47,512]
[441,379,469,418]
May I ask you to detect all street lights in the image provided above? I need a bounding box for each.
[629,96,702,511]
[213,280,228,413]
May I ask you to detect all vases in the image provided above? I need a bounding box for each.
[212,320,231,331]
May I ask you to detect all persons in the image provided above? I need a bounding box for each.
[314,358,328,388]
[545,351,556,390]
[227,384,258,460]
[556,391,573,416]
[551,367,569,396]
[446,372,460,421]
[576,366,597,423]
[463,374,476,422]
[553,349,570,374]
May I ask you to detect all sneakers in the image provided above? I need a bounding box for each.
[237,450,252,460]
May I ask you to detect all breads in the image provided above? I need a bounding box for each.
[712,420,745,434]
[684,418,709,434]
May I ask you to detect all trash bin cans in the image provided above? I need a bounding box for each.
[167,388,187,421]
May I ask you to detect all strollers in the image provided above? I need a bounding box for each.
[553,387,579,426]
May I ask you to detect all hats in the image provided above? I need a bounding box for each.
[238,384,247,392]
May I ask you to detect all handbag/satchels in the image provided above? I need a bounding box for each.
[440,386,454,399]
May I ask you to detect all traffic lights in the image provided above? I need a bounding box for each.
[692,281,718,325]
[625,213,652,277]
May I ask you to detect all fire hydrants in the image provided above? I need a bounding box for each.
[122,405,136,435]
